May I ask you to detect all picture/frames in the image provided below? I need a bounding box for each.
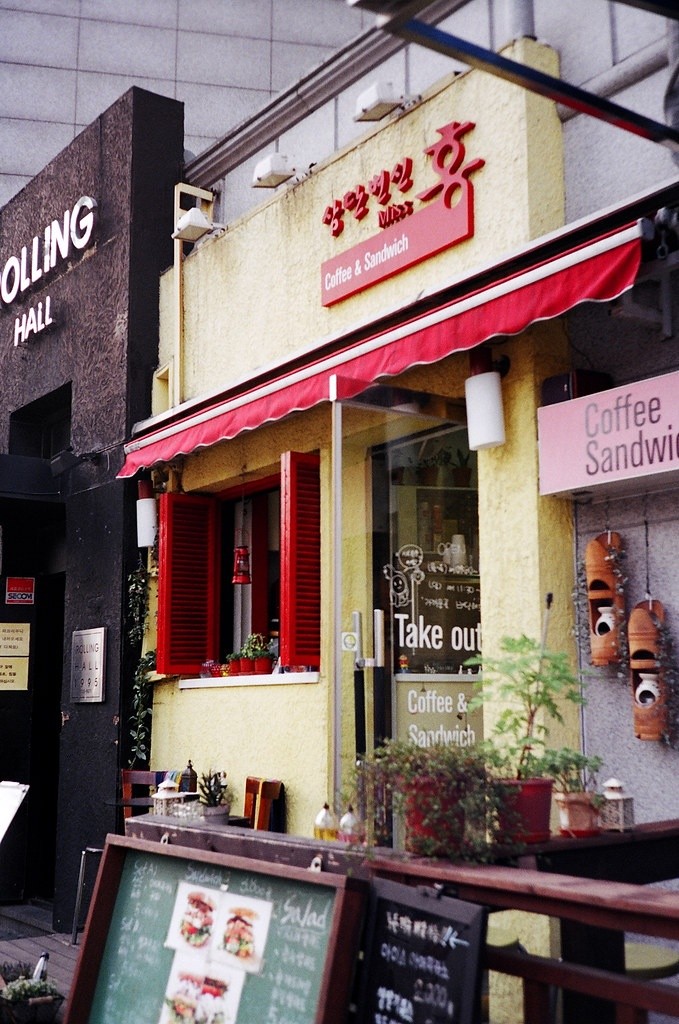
[69,627,106,704]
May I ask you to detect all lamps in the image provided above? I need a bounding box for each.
[170,209,229,242]
[465,372,506,451]
[232,463,252,584]
[353,79,423,124]
[250,153,310,188]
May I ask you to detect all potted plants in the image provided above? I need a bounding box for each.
[226,652,240,675]
[334,739,510,872]
[0,976,65,1024]
[463,592,591,842]
[533,749,605,835]
[197,768,240,826]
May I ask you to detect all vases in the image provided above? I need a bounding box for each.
[255,657,272,674]
[240,656,254,674]
[595,606,615,635]
[628,601,679,750]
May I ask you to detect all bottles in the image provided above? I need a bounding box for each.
[339,804,360,847]
[33,952,49,982]
[314,802,335,840]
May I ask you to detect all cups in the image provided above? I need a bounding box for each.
[450,535,468,570]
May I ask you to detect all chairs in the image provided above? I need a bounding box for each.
[244,776,282,830]
[70,770,169,944]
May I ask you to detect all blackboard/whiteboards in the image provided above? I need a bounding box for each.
[344,876,488,1024]
[61,831,369,1024]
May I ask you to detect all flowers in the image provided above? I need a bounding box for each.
[654,618,677,750]
[574,547,629,687]
[241,632,274,658]
